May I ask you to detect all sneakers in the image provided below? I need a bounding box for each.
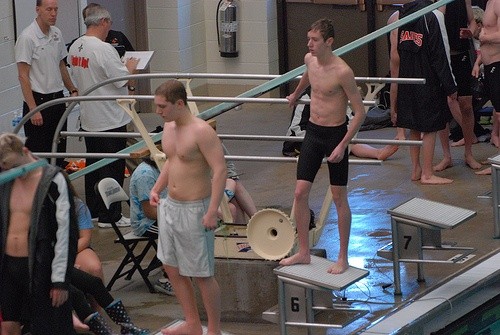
[97,213,131,228]
[154,278,176,296]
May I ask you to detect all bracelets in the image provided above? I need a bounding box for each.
[69,89,77,94]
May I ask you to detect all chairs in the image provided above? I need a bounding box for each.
[94,177,160,293]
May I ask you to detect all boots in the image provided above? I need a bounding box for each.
[84,312,118,335]
[105,299,151,335]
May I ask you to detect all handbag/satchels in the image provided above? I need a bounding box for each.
[379,71,391,110]
[359,104,391,130]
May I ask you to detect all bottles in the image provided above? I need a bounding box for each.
[11,110,25,139]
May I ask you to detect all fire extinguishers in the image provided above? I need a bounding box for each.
[216,0,240,58]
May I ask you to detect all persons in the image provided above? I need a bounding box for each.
[277,19,366,274]
[282,84,398,161]
[0,0,258,335]
[386,0,500,184]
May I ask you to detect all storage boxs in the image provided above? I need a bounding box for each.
[214,222,264,259]
